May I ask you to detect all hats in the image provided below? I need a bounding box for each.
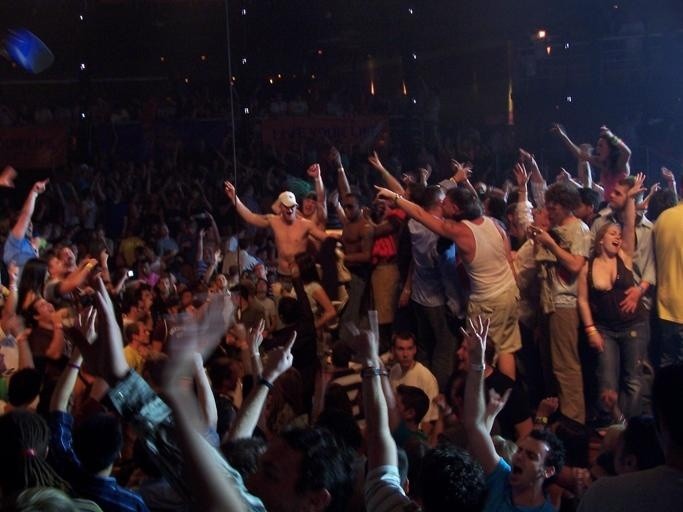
[278,191,299,209]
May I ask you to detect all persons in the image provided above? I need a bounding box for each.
[0,65,682,512]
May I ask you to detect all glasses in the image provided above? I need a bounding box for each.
[341,202,355,210]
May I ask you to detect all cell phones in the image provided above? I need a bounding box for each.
[127,271,134,276]
